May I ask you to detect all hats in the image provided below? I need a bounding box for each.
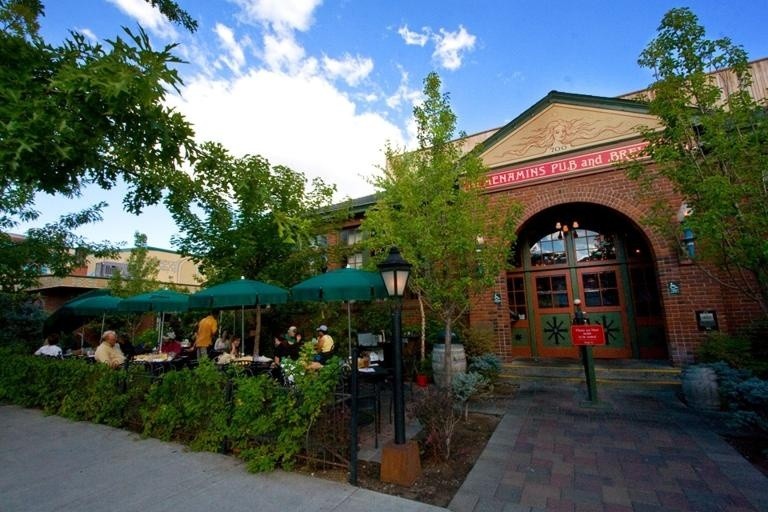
[316,325,328,331]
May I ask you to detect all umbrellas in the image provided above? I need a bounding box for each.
[63,287,190,353]
[290,266,388,357]
[190,277,289,360]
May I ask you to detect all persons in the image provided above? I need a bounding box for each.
[499,118,641,157]
[195,308,218,364]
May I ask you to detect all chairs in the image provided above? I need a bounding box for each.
[357,355,416,450]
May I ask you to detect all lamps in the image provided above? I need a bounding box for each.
[675,220,697,266]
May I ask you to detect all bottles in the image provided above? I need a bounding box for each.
[347,356,352,371]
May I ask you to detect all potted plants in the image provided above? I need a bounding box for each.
[410,359,432,385]
